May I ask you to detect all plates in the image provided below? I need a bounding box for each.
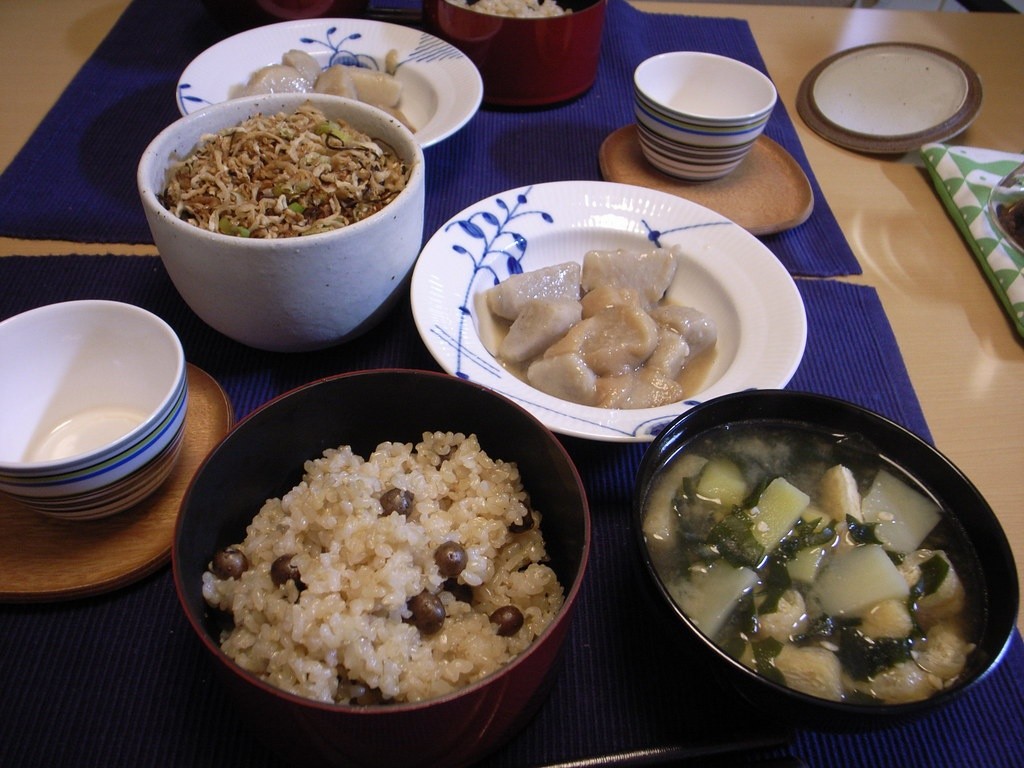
[410,178,808,441]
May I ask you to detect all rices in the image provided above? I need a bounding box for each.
[202,426,565,705]
[449,0,574,18]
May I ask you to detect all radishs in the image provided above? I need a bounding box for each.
[641,449,945,641]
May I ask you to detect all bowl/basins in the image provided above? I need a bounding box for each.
[135,92,422,352]
[174,368,592,768]
[631,390,1020,726]
[420,0,607,108]
[632,50,778,182]
[175,17,485,161]
[0,299,189,520]
